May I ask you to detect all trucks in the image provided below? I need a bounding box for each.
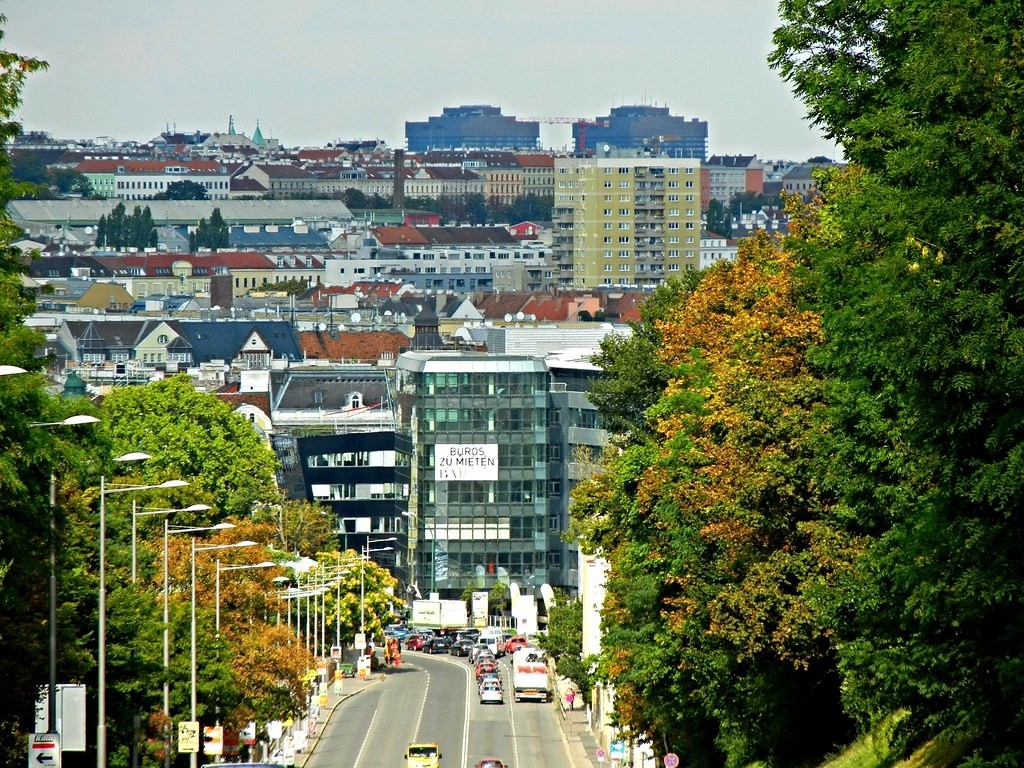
[510,648,548,703]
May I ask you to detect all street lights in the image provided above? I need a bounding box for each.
[96,475,190,768]
[191,537,256,768]
[131,501,215,768]
[215,559,277,761]
[165,519,236,768]
[402,511,434,592]
[249,536,397,754]
[46,453,151,733]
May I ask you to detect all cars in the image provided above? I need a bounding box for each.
[404,634,429,651]
[384,630,408,640]
[339,663,355,677]
[475,757,508,768]
[418,627,502,641]
[474,649,505,705]
[404,743,442,768]
[449,640,475,657]
[478,634,506,659]
[505,637,528,655]
[421,638,449,654]
